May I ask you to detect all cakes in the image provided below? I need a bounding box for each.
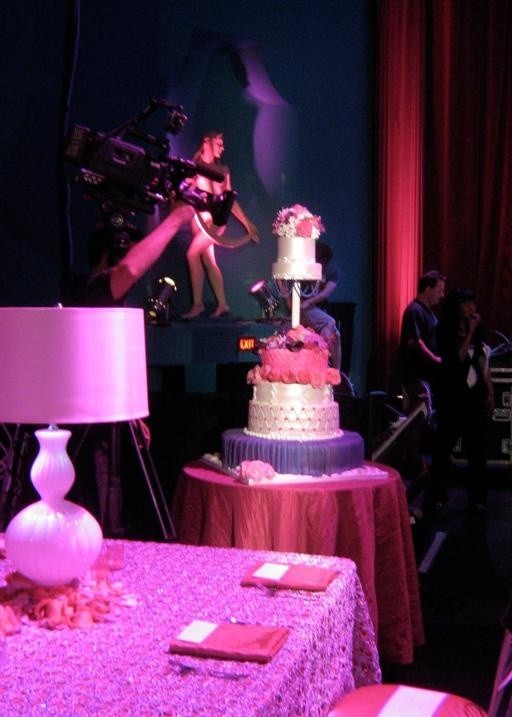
[270,203,325,283]
[221,326,363,474]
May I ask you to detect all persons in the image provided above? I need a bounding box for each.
[438,288,493,474]
[275,239,341,368]
[63,203,196,540]
[107,131,261,321]
[396,269,444,418]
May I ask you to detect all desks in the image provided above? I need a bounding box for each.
[173,466,425,664]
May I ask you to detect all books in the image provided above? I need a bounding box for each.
[166,618,293,664]
[238,558,343,591]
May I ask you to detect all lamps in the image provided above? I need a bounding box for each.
[0,303,149,589]
[248,279,284,327]
[147,277,177,326]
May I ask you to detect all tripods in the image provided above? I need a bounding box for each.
[41,392,184,547]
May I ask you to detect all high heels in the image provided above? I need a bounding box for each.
[180,302,206,320]
[209,304,231,318]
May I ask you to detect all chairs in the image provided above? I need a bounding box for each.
[326,629,512,717]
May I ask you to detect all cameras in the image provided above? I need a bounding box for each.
[66,99,237,243]
